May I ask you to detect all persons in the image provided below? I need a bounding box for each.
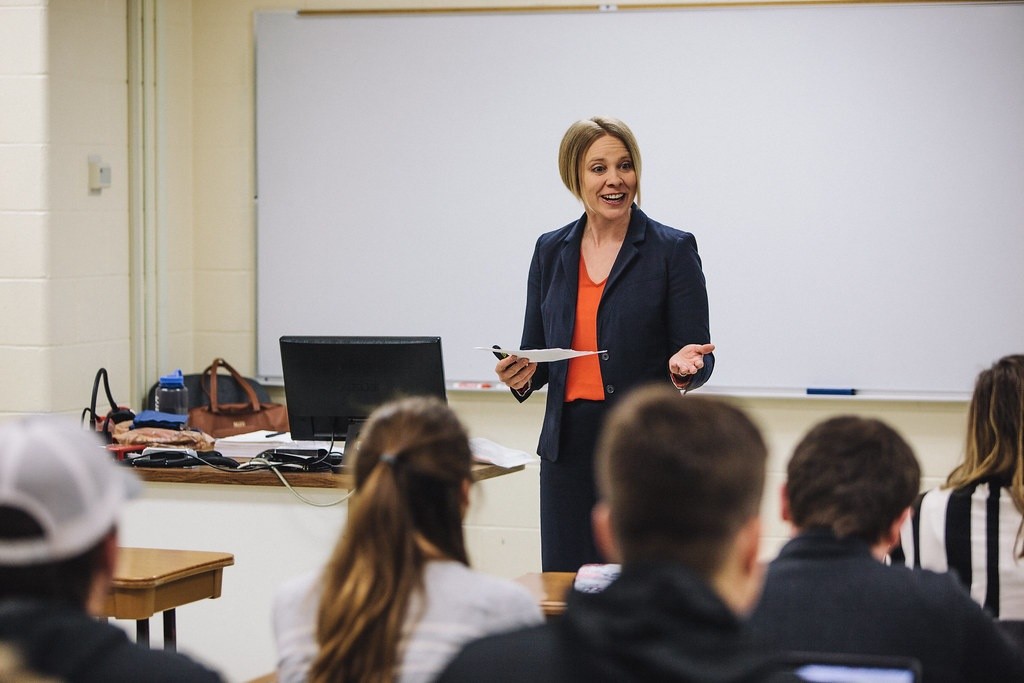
[281,395,546,683]
[428,386,806,683]
[747,416,1020,683]
[879,355,1020,621]
[0,416,225,683]
[495,116,716,572]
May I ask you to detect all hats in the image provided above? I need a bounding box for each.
[0,418,142,564]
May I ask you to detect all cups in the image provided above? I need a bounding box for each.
[155,369,188,415]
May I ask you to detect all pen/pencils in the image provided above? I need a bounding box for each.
[265,431,286,438]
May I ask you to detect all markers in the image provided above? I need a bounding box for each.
[491,344,523,373]
[454,383,492,389]
[806,387,854,395]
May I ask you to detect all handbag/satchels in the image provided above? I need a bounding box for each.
[188,358,290,438]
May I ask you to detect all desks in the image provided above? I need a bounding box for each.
[117,453,524,682]
[100,547,235,651]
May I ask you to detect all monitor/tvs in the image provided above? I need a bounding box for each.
[279,335,448,474]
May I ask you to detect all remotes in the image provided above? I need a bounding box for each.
[493,345,517,369]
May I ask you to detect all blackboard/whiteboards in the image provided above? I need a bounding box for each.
[250,0,1024,405]
